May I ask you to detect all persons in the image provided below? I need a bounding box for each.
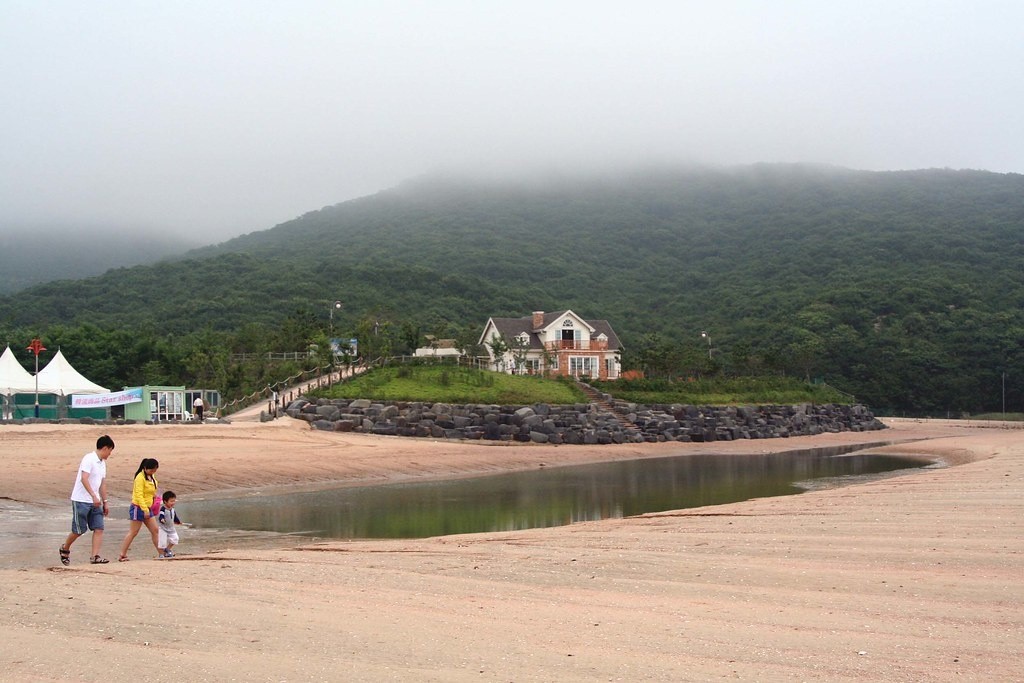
[193,397,204,420]
[157,491,182,558]
[59,435,115,566]
[118,458,166,562]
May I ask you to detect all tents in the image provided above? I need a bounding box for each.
[33,350,110,421]
[0,347,34,420]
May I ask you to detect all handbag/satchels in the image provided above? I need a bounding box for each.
[150,497,162,515]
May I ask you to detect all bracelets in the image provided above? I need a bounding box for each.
[104,500,108,502]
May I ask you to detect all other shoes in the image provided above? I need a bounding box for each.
[119,554,129,562]
[165,548,173,557]
[159,553,164,558]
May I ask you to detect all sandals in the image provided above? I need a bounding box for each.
[89,555,109,564]
[59,544,70,566]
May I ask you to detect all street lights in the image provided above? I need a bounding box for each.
[702,331,711,358]
[26,338,42,418]
[330,299,342,337]
